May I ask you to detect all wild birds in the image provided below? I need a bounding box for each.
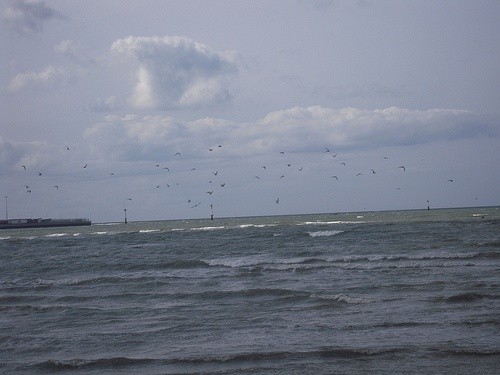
[21,144,478,205]
